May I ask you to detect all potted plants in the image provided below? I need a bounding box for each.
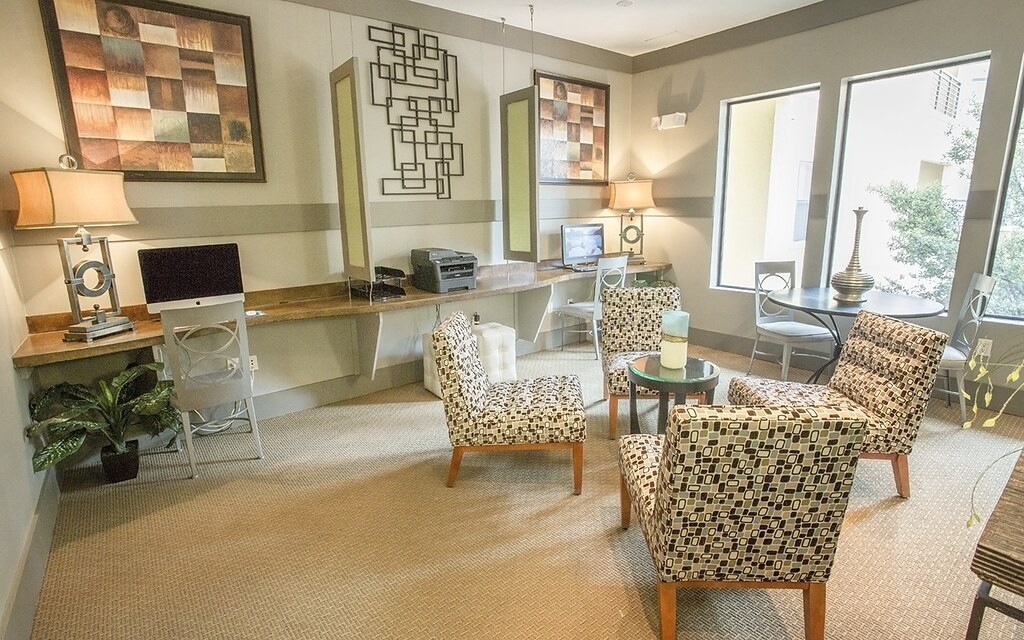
[24,361,184,486]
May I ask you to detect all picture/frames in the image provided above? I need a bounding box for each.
[37,0,268,185]
[533,68,610,186]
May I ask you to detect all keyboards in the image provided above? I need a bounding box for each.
[573,266,597,272]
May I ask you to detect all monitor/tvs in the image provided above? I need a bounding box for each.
[561,223,605,268]
[137,243,246,314]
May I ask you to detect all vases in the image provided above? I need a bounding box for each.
[830,206,875,304]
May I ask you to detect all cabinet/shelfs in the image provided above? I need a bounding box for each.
[350,266,408,301]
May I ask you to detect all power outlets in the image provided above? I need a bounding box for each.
[976,338,994,356]
[227,359,241,370]
[249,355,259,371]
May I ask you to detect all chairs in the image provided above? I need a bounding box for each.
[728,308,953,499]
[160,301,265,479]
[561,255,628,361]
[938,273,995,424]
[601,286,707,441]
[433,312,589,495]
[745,261,840,385]
[620,404,866,640]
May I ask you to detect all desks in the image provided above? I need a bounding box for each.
[626,353,721,436]
[964,447,1024,639]
[769,288,944,382]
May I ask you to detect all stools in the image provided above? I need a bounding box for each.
[424,321,514,398]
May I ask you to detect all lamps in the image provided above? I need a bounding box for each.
[9,154,140,343]
[608,172,657,265]
